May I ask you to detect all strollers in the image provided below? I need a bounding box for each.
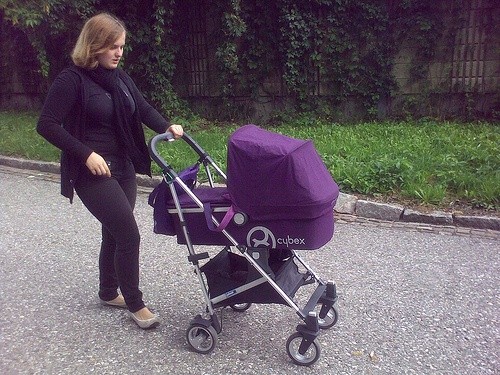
[148,124,336,365]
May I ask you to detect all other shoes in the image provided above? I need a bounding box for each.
[128,306,160,329]
[101,295,129,307]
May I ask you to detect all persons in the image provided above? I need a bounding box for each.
[38,14,184,329]
